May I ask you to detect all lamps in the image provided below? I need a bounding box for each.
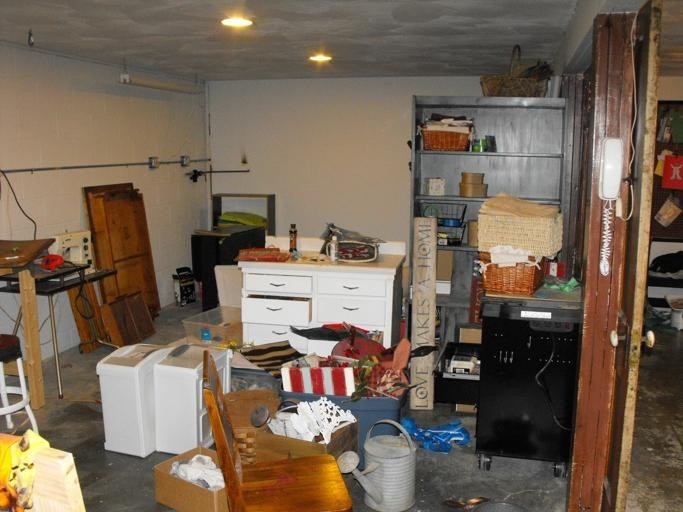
[120,74,202,93]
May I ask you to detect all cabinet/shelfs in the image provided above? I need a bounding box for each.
[647,100,683,308]
[406,95,575,348]
[238,236,407,359]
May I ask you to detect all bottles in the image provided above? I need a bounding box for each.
[199,326,210,345]
[326,236,339,261]
[288,223,297,257]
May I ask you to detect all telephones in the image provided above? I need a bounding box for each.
[598,138,624,203]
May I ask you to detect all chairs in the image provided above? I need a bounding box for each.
[202,350,353,512]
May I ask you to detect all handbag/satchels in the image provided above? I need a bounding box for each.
[320,223,385,262]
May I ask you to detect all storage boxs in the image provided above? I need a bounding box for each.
[153,446,229,512]
[256,415,359,463]
[181,306,241,352]
[279,390,409,470]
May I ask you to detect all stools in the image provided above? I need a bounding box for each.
[0,334,39,434]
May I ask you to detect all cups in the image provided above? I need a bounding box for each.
[472,138,487,152]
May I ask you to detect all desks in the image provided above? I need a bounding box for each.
[0,263,120,399]
[0,238,55,410]
